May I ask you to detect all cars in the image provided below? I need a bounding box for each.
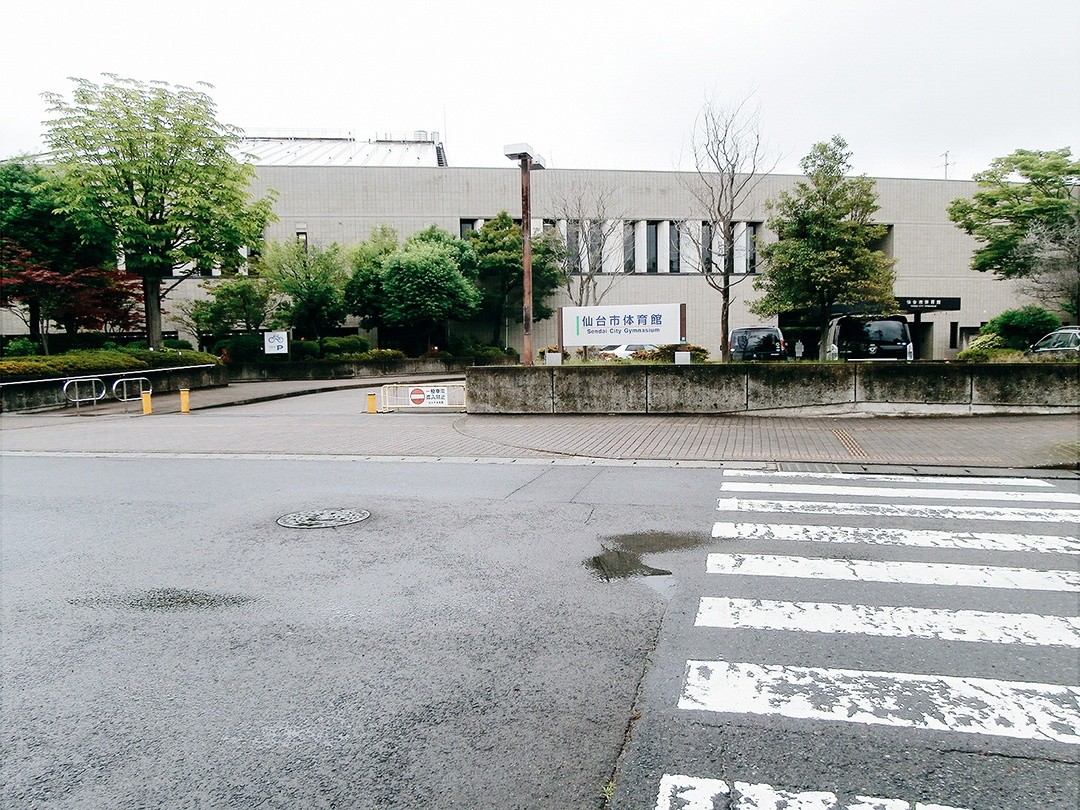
[599,344,661,361]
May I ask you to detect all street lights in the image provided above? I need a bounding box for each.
[504,142,549,365]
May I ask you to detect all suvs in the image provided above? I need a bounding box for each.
[1021,326,1080,358]
[818,314,915,362]
[720,325,787,362]
